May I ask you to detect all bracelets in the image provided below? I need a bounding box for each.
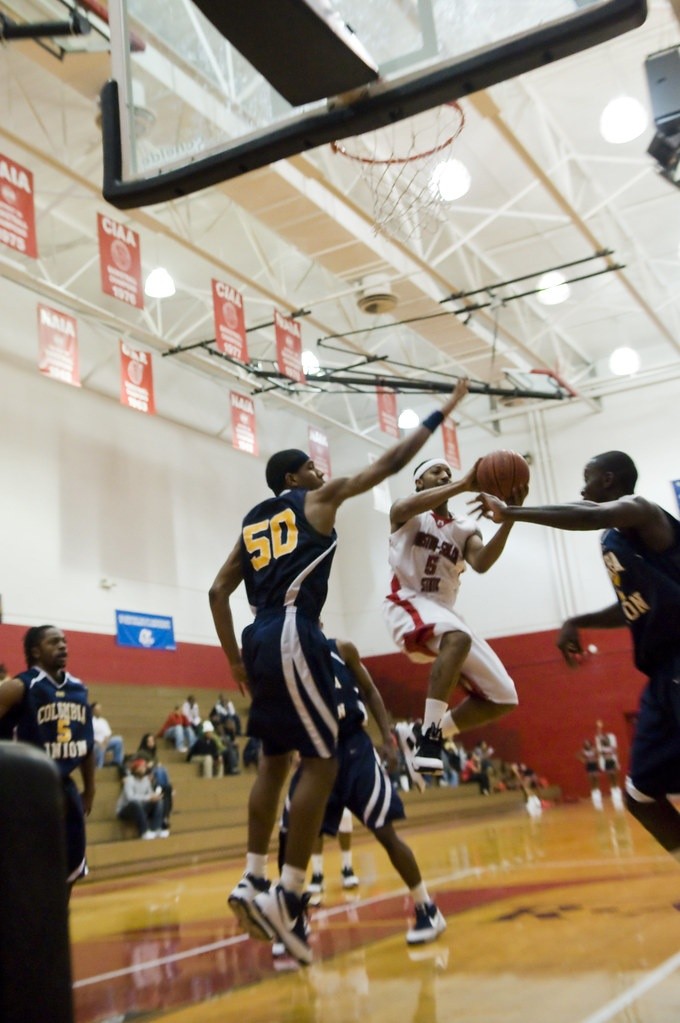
[423,411,444,433]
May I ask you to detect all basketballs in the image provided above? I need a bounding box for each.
[474,448,530,504]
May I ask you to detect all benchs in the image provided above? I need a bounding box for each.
[71,681,561,886]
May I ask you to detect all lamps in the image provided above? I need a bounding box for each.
[144,266,175,299]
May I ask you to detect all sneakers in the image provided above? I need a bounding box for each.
[227,872,276,941]
[248,882,313,965]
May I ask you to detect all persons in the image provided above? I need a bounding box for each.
[91,702,174,839]
[209,377,469,964]
[271,617,446,955]
[578,720,625,812]
[0,741,75,1023]
[157,694,261,779]
[382,717,543,816]
[465,451,680,911]
[384,458,530,796]
[0,625,96,901]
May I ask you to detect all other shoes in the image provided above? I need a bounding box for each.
[340,866,358,888]
[406,902,446,945]
[390,719,451,794]
[307,874,324,905]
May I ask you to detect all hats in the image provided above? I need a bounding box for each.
[201,720,214,733]
[130,759,144,774]
